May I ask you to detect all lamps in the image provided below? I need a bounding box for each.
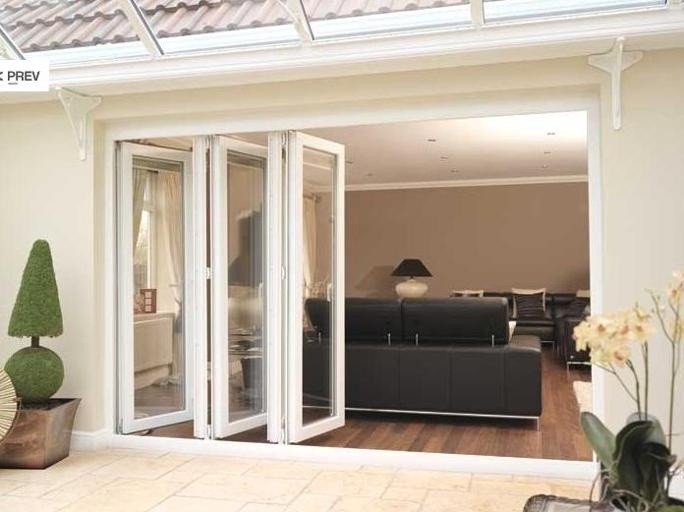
[389,258,433,297]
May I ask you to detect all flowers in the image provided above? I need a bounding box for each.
[570,269,683,512]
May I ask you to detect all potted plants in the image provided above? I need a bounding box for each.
[0,239,83,470]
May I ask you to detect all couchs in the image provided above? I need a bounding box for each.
[451,289,591,372]
[301,297,542,420]
